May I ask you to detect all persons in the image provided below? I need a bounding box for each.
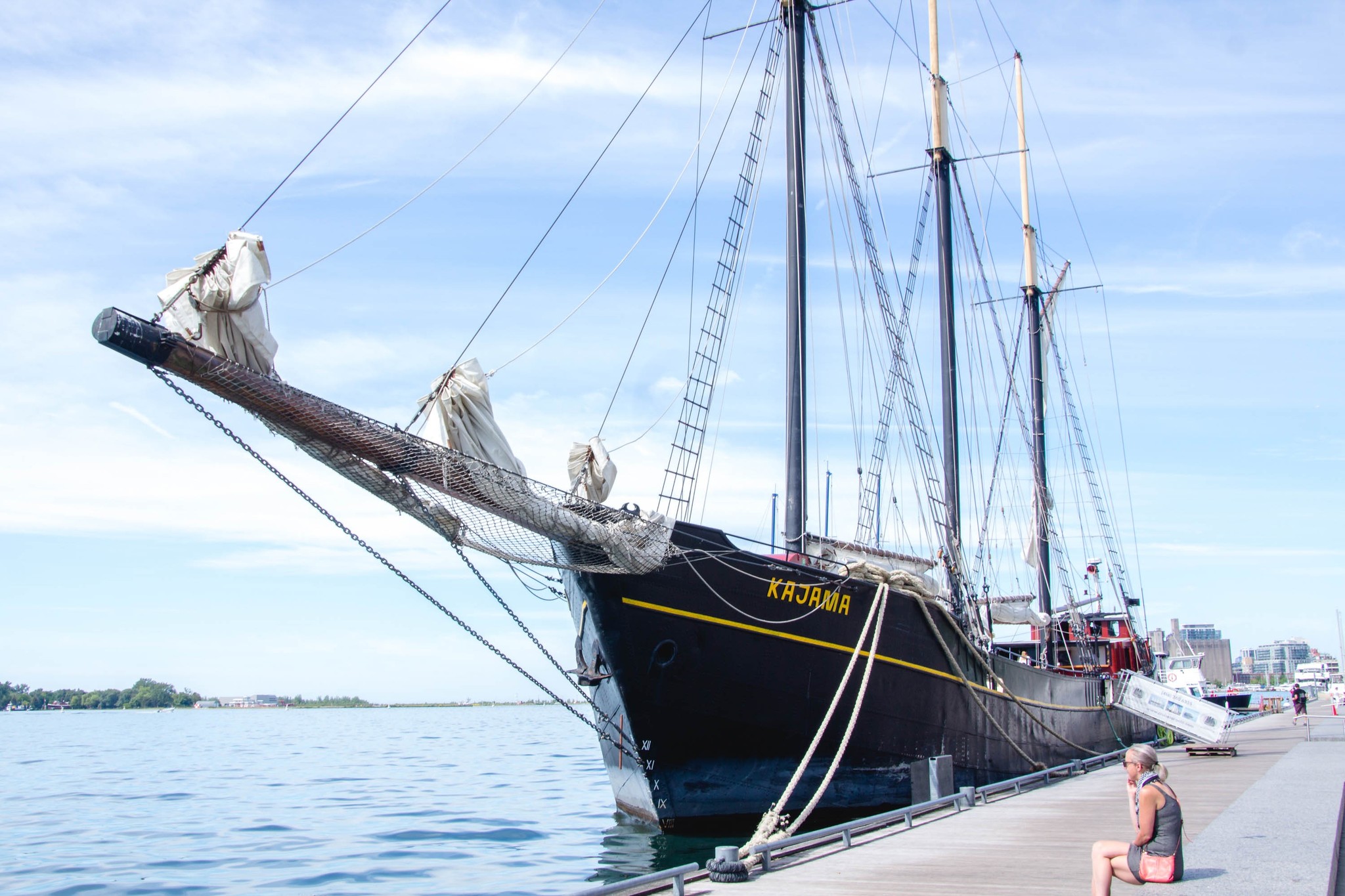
[1018,651,1031,667]
[1227,687,1234,696]
[1334,686,1345,710]
[1289,684,1308,726]
[1210,691,1216,697]
[1091,743,1184,896]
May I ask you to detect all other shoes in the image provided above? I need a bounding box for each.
[1292,721,1296,725]
[1303,722,1308,725]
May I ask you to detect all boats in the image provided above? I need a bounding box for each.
[1156,635,1253,708]
[1294,662,1333,692]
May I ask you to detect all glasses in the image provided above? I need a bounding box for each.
[1122,760,1145,768]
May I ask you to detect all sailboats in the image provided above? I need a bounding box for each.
[89,0,1158,839]
